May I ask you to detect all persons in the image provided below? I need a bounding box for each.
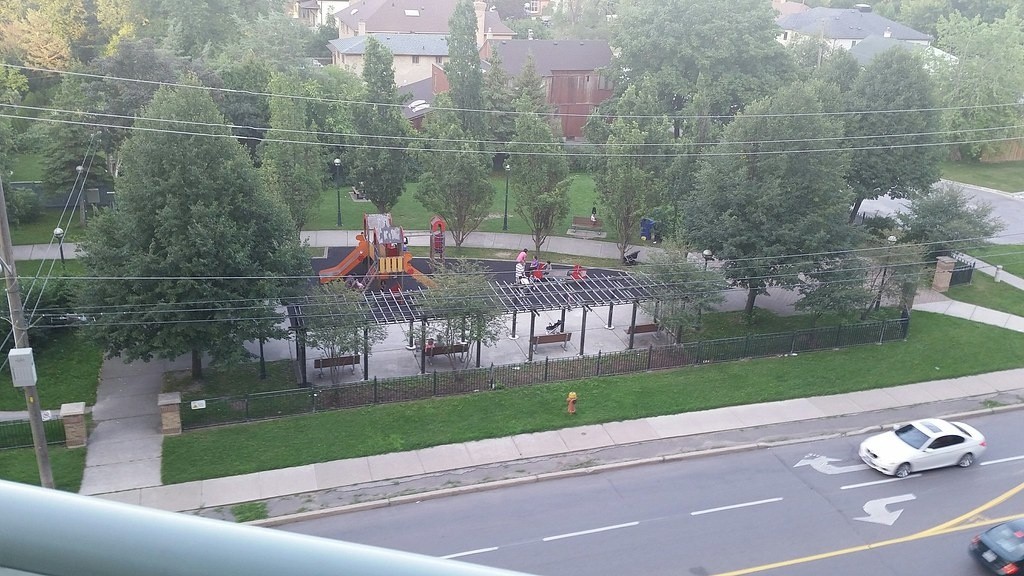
[542,261,552,275]
[426,338,435,350]
[531,255,538,269]
[434,334,445,348]
[516,249,528,262]
[346,272,357,285]
[516,260,526,281]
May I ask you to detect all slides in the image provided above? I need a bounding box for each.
[319,241,369,284]
[403,251,437,287]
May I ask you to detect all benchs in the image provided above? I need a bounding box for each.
[529,332,571,354]
[352,186,363,199]
[571,217,603,235]
[624,323,660,340]
[314,355,360,378]
[426,344,469,366]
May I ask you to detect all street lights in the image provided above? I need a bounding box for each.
[694,249,712,328]
[75,165,90,229]
[53,227,66,270]
[873,235,897,313]
[333,158,343,226]
[503,164,512,231]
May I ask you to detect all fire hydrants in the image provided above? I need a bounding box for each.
[566,391,577,415]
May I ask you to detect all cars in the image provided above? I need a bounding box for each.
[858,417,987,481]
[966,515,1024,576]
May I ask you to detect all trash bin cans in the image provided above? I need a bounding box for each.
[640,218,656,241]
[653,222,662,242]
